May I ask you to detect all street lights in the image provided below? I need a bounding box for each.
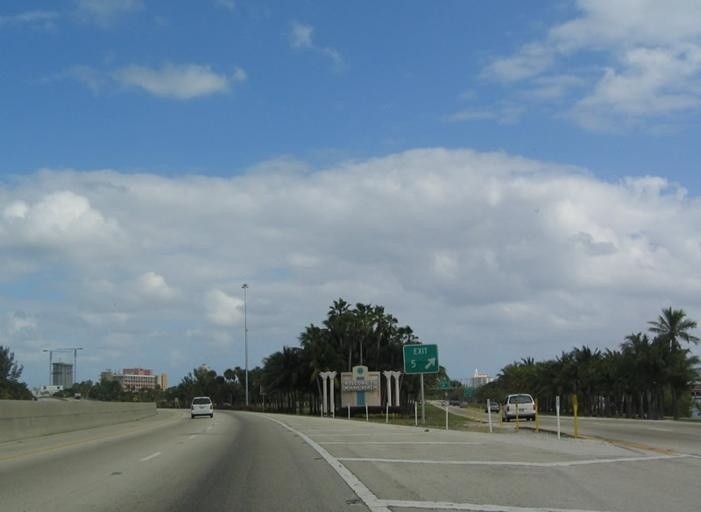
[42,350,52,386]
[73,347,83,384]
[241,282,252,405]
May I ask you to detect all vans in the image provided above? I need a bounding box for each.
[501,394,536,422]
[190,395,213,419]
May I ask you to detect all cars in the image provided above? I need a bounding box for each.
[460,402,467,408]
[441,400,450,406]
[484,401,500,413]
[74,393,80,399]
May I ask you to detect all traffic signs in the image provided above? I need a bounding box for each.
[402,342,439,376]
